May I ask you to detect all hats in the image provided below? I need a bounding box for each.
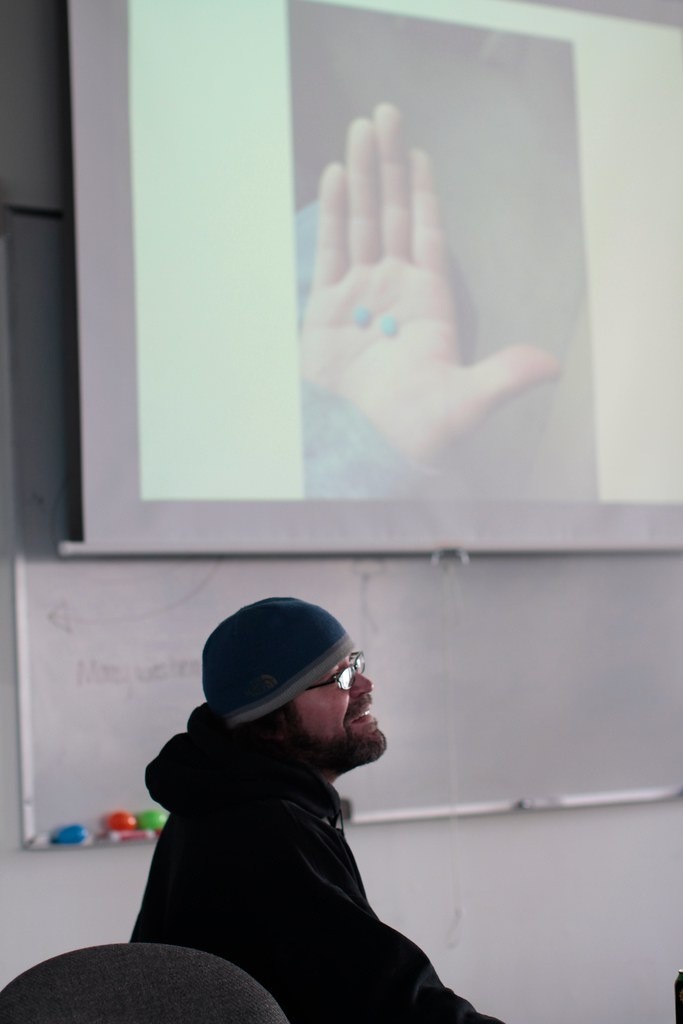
[201,594,353,727]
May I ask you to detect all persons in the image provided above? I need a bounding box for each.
[305,101,559,471]
[129,596,504,1024]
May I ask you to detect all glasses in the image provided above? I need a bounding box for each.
[303,651,366,691]
